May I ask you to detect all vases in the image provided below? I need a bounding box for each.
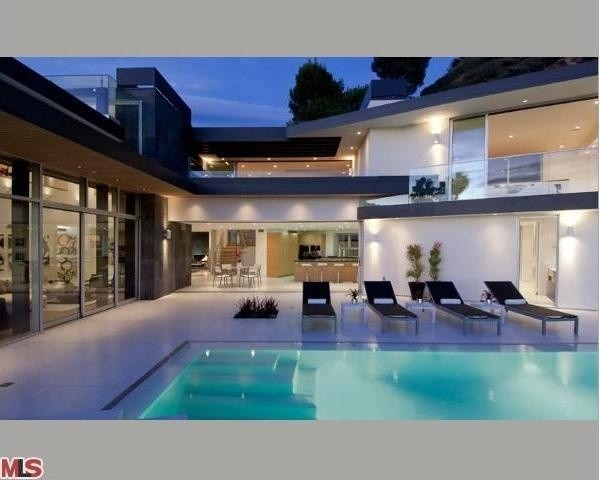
[352,298,357,304]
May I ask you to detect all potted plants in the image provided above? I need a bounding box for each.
[406,244,425,300]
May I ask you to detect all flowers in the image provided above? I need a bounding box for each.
[347,288,362,299]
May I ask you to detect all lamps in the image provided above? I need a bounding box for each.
[566,225,575,240]
[430,133,441,146]
[370,231,379,244]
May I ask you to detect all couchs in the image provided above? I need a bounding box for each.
[0,279,47,322]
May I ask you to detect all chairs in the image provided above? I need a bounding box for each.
[212,262,262,288]
[363,281,419,335]
[426,281,501,336]
[483,281,578,336]
[301,282,337,333]
